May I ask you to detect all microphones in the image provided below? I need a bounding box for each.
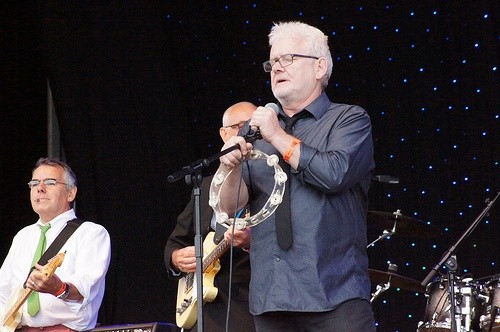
[247,102,279,137]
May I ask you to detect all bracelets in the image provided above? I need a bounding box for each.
[54,283,66,298]
[282,137,302,164]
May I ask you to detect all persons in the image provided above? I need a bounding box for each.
[162,101,257,332]
[0,157,111,332]
[209,20,380,332]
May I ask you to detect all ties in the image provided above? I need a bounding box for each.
[275,109,310,251]
[28,224,51,317]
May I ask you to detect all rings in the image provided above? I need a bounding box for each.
[30,282,36,286]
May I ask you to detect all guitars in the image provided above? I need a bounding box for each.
[3,253,65,332]
[176,211,250,330]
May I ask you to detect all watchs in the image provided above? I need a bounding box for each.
[55,283,70,299]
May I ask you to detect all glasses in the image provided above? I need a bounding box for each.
[28,178,71,189]
[225,120,246,132]
[262,53,319,74]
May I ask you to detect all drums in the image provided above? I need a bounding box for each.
[479,274,500,332]
[417,278,481,332]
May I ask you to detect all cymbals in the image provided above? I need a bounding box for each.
[208,150,288,229]
[366,211,447,240]
[368,268,428,293]
